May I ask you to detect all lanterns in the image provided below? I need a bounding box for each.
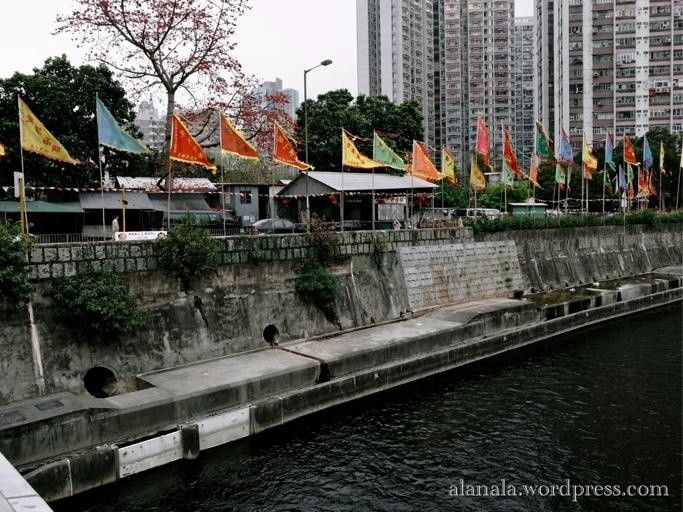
[281,199,288,208]
[328,194,338,203]
[415,195,425,206]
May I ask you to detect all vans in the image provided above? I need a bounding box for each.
[161,210,239,238]
[450,207,503,223]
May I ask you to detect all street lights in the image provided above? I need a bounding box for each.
[303,59,335,231]
[583,139,606,215]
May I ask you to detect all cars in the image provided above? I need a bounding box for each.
[243,218,294,236]
[327,220,362,231]
[13,232,39,244]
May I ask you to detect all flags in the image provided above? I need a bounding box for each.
[97,95,155,155]
[168,110,217,176]
[414,106,682,199]
[372,127,408,173]
[19,96,81,167]
[340,128,390,171]
[272,119,315,171]
[220,108,261,165]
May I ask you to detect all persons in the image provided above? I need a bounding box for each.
[111,215,119,241]
